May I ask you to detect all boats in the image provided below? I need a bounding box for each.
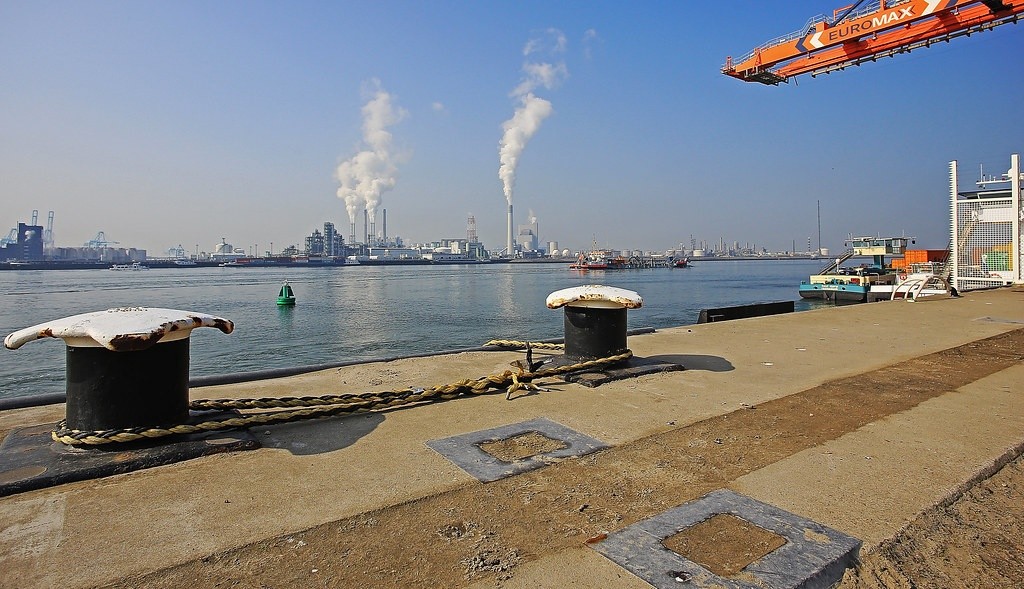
[218,261,246,267]
[109,261,150,272]
[343,258,361,266]
